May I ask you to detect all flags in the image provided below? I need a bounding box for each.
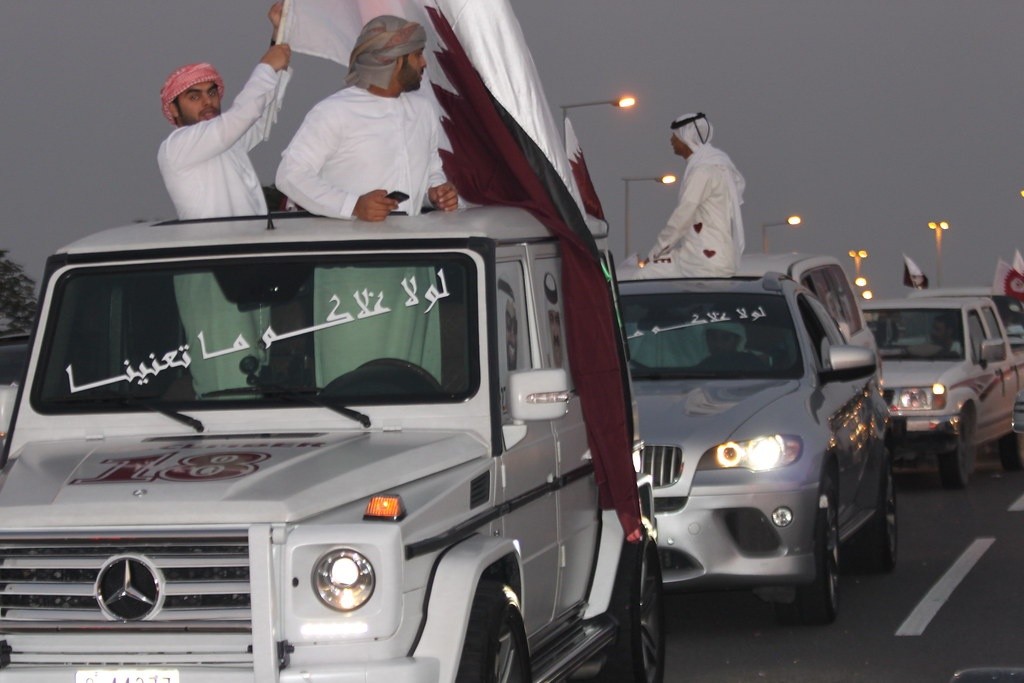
[275,0,651,545]
[991,248,1024,300]
[900,251,929,290]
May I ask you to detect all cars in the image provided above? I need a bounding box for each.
[729,247,887,402]
[600,272,898,624]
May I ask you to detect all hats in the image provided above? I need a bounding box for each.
[344,15,426,90]
[160,63,224,125]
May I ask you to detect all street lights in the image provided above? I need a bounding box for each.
[765,215,802,260]
[622,175,677,263]
[849,250,867,300]
[928,220,949,289]
[559,95,637,167]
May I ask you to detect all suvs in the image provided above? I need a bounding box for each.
[858,287,1024,488]
[0,206,668,683]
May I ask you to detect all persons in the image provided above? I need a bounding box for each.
[626,110,773,379]
[154,0,292,405]
[927,312,964,357]
[274,14,467,392]
